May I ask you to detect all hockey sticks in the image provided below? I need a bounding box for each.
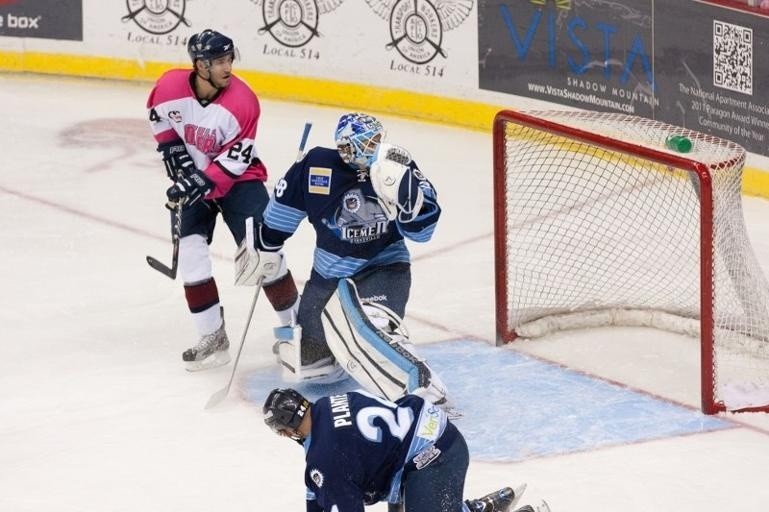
[146,169,184,279]
[205,124,311,409]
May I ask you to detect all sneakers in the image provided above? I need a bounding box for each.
[515,504,535,512]
[182,330,230,360]
[482,487,514,512]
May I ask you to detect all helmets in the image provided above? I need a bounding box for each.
[187,29,234,66]
[336,113,384,170]
[263,388,306,444]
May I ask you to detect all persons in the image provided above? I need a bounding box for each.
[263,388,536,512]
[252,114,444,405]
[144,24,303,360]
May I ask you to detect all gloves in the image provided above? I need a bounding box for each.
[158,137,197,182]
[165,170,214,212]
